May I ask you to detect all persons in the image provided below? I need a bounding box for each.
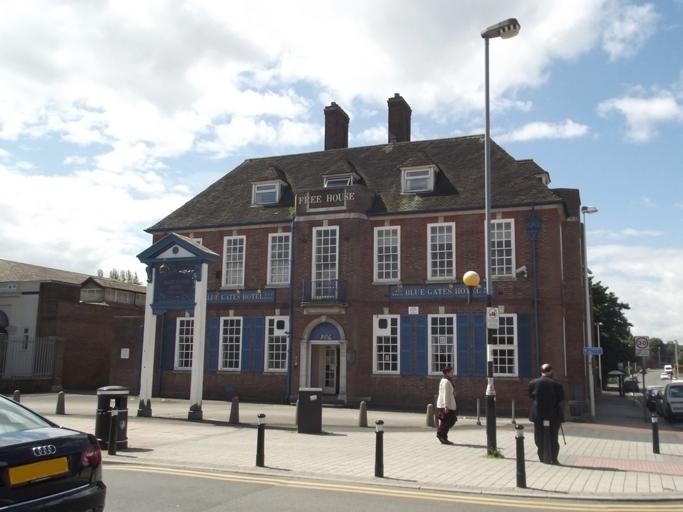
[527,362,564,465]
[434,365,458,444]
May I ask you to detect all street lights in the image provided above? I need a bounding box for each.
[582,206,598,416]
[481,18,520,453]
[594,322,603,392]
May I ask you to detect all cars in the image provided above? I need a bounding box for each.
[0,394,106,512]
[622,365,683,424]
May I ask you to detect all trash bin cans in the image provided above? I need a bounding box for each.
[96,384,127,450]
[298,387,322,434]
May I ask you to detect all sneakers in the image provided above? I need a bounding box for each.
[436,434,450,445]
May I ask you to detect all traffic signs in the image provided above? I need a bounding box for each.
[635,336,649,356]
